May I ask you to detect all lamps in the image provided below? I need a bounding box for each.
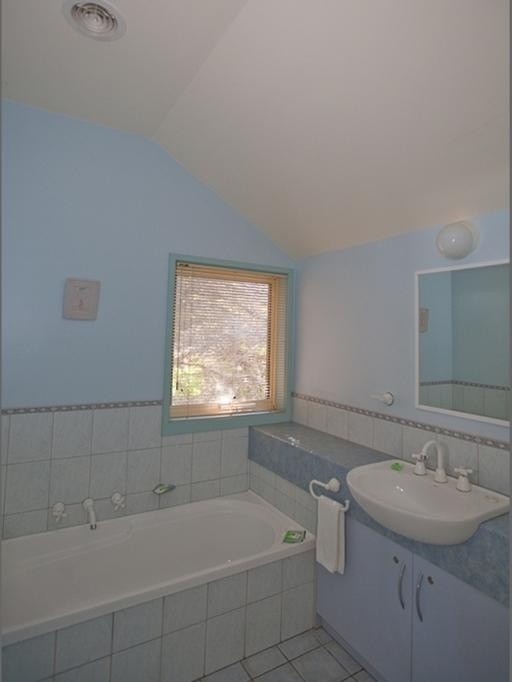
[436,222,477,261]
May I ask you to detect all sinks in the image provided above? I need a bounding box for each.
[347,459,511,546]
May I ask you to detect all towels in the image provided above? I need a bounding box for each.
[316,494,345,575]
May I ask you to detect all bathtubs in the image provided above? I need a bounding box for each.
[1,488,319,681]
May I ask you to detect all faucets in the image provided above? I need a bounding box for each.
[412,439,446,482]
[83,497,98,530]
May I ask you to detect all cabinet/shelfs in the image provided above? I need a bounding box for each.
[312,516,511,682]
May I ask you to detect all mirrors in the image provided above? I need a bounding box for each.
[414,260,511,429]
